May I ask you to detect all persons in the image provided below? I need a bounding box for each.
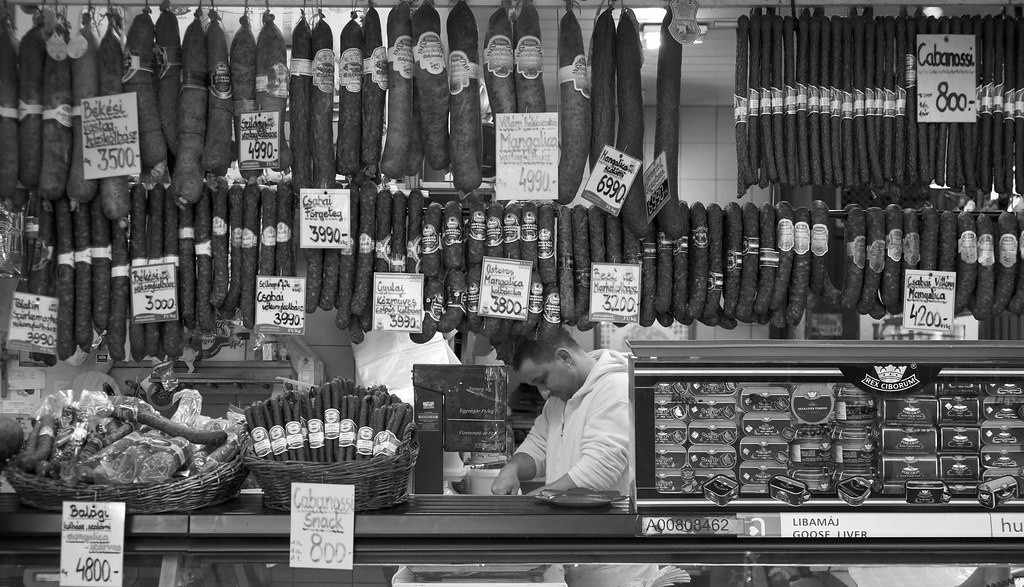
[492,324,636,502]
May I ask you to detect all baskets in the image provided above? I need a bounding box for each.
[5,431,248,514]
[237,421,420,511]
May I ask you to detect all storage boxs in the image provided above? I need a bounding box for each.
[4,423,250,513]
[238,423,420,513]
[653,383,1024,498]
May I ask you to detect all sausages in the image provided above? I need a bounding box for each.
[0,0,1024,360]
[16,373,414,486]
